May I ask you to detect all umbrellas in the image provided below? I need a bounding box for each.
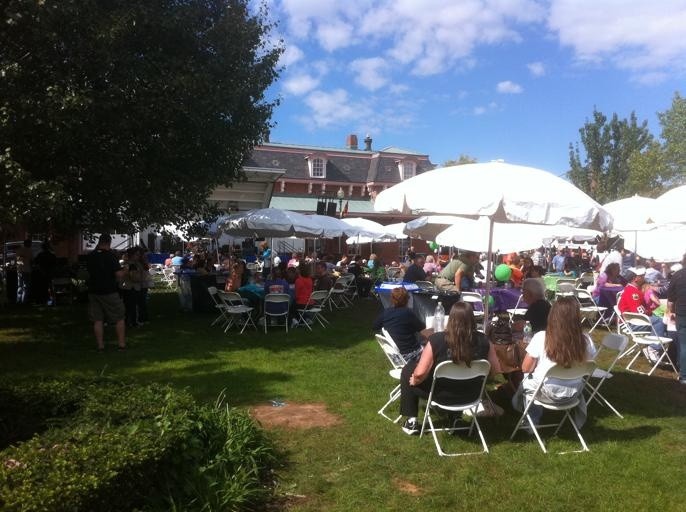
[644,185,686,227]
[594,192,686,260]
[371,159,617,334]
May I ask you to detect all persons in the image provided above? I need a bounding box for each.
[519,296,597,435]
[510,299,553,391]
[15,207,685,387]
[401,300,502,436]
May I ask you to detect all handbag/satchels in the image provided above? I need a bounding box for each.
[490,322,513,345]
[477,399,496,417]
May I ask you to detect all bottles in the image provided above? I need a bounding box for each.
[522,320,532,343]
[433,301,445,332]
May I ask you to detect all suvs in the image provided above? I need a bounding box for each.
[0,240,44,265]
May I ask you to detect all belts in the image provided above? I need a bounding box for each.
[438,276,451,281]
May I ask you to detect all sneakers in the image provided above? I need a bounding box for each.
[96,345,105,354]
[117,345,128,353]
[642,347,652,363]
[401,418,419,436]
[677,376,686,384]
[447,417,464,435]
[291,319,299,328]
[647,346,662,366]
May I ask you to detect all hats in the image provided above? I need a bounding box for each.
[626,266,646,281]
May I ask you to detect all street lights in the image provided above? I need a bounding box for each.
[337,187,345,254]
